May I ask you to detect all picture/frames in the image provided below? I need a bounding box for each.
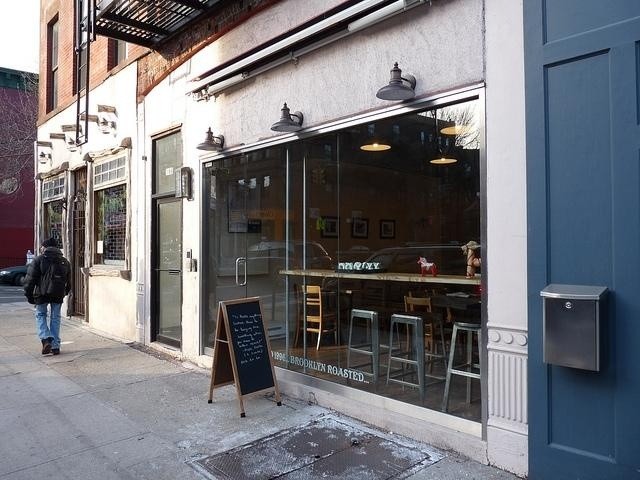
[379,219,395,239]
[352,217,369,239]
[319,215,341,238]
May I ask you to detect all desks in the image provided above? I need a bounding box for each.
[278,268,480,286]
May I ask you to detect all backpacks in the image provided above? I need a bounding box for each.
[41,253,68,296]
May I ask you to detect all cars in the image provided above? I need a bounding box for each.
[242,242,481,321]
[0,265,28,286]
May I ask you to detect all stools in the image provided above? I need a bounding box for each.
[346,306,404,384]
[386,311,448,395]
[441,318,481,413]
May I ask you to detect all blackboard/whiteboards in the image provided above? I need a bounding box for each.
[220,296,277,399]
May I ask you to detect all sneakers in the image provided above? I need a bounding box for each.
[52,349,59,355]
[42,340,51,354]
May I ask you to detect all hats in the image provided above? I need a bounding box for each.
[41,238,58,248]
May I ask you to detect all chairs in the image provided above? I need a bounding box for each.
[403,289,455,370]
[295,284,337,351]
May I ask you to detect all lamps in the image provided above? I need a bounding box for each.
[359,134,391,152]
[439,119,472,136]
[96,117,116,134]
[376,62,416,100]
[66,138,80,152]
[196,126,225,151]
[39,151,51,164]
[270,102,304,132]
[429,136,458,165]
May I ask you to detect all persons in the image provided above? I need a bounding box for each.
[23,238,71,354]
